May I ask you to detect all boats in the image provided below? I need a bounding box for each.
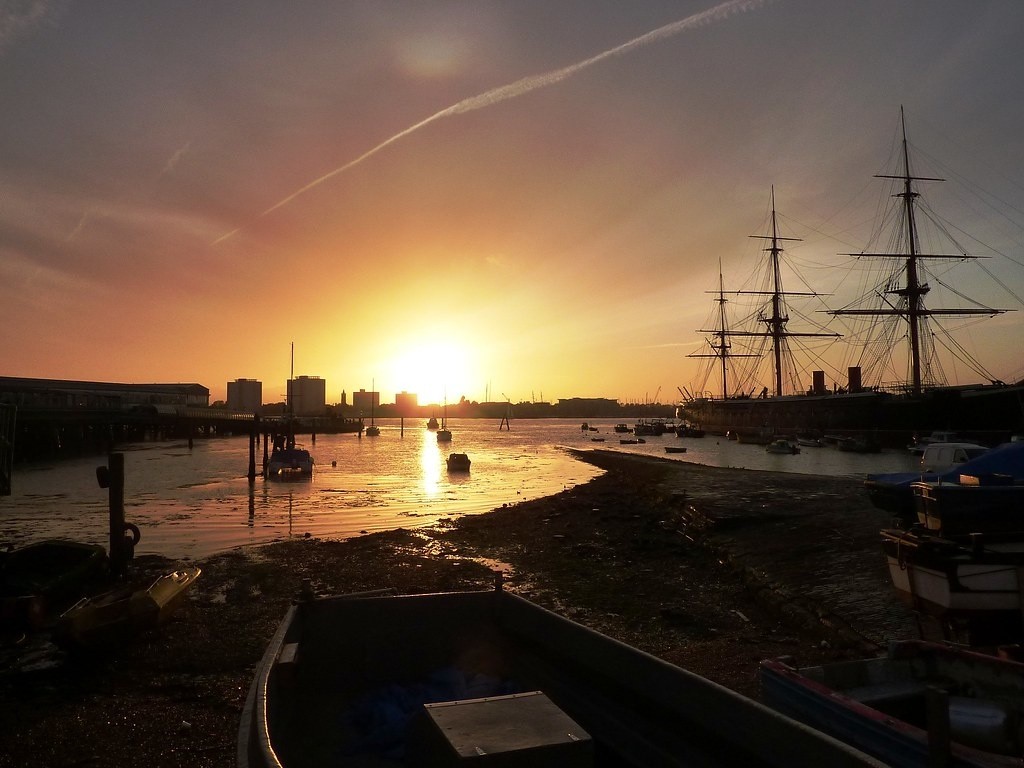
[673,425,705,439]
[726,426,856,447]
[437,431,453,441]
[909,474,1024,535]
[634,418,674,436]
[614,423,634,432]
[621,440,638,444]
[879,526,1024,610]
[589,427,597,431]
[447,454,471,471]
[427,418,438,429]
[591,438,604,442]
[838,440,868,452]
[756,633,1023,768]
[581,423,588,430]
[666,447,687,453]
[239,584,888,768]
[765,440,801,454]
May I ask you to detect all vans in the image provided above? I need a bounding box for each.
[919,442,991,476]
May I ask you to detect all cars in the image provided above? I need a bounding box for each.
[908,431,961,455]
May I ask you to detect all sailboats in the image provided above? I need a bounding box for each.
[674,104,1023,443]
[267,346,313,478]
[364,392,380,436]
